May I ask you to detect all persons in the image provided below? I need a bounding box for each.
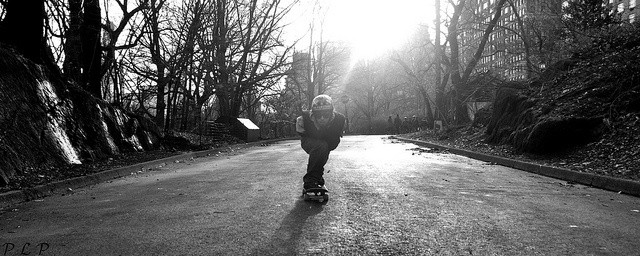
[387,113,429,135]
[295,95,346,190]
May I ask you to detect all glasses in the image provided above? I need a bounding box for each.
[315,112,331,118]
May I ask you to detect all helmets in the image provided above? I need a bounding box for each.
[311,94,334,112]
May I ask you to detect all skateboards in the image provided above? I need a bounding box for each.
[302,183,329,202]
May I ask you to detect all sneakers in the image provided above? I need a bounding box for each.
[304,177,324,188]
